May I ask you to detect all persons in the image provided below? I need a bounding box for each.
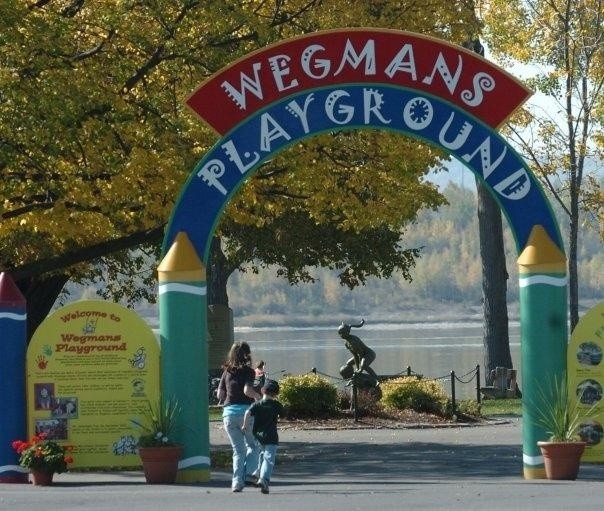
[338,325,378,387]
[217,341,286,496]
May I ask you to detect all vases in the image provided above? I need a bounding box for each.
[139,444,183,483]
[29,464,56,485]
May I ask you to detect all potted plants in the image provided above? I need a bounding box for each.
[513,371,602,479]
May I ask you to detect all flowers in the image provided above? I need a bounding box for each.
[11,432,73,474]
[124,391,183,447]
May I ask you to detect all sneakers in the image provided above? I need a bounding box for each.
[232,470,269,494]
